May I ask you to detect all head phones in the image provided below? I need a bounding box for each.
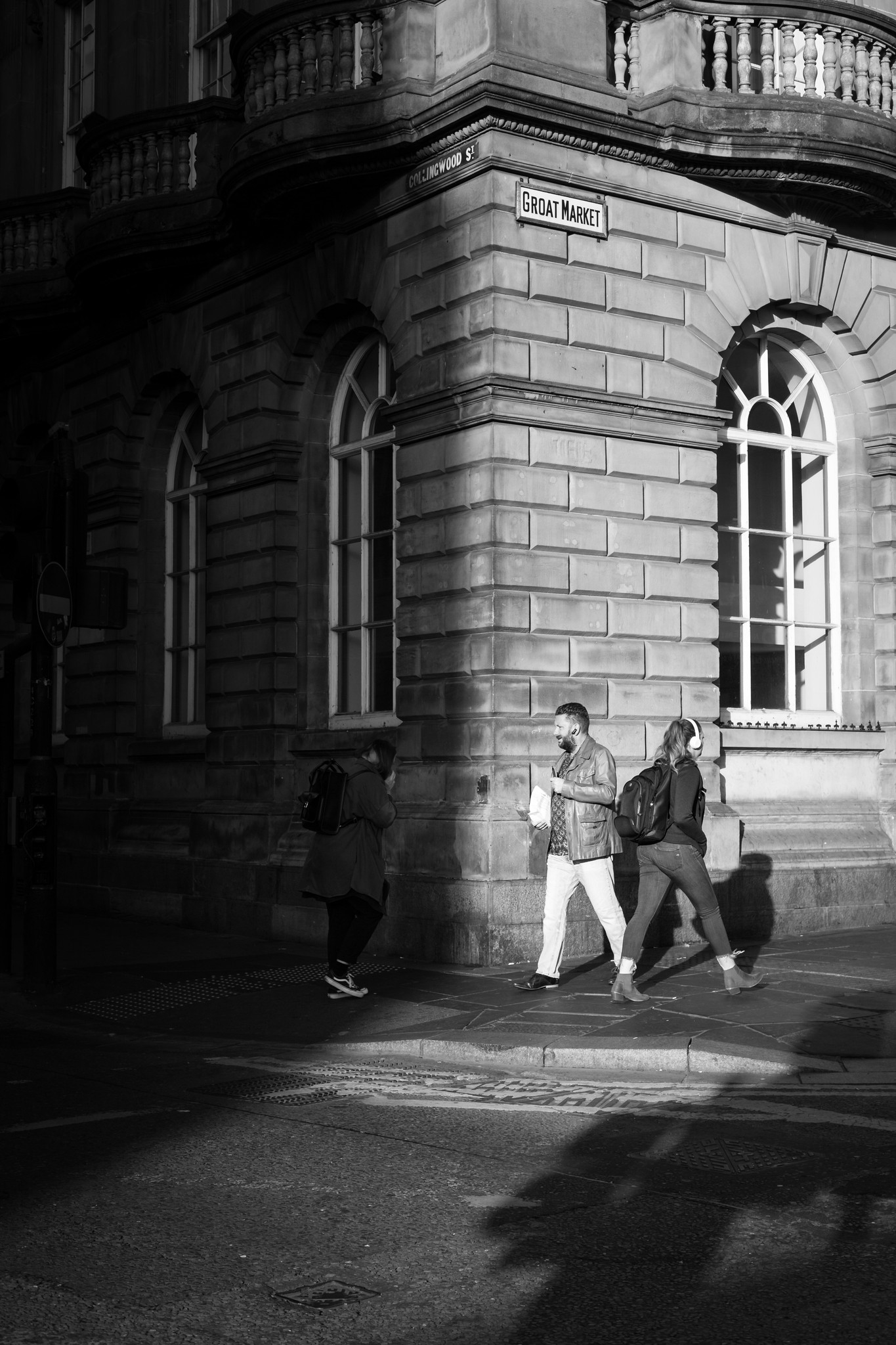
[685,718,701,749]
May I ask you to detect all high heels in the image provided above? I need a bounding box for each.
[723,964,764,995]
[611,973,650,1001]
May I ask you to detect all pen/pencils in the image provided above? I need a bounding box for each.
[552,766,556,777]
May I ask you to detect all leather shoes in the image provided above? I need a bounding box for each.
[513,974,559,990]
[608,968,636,984]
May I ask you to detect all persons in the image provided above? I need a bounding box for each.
[611,717,764,1002]
[296,738,398,999]
[514,703,637,991]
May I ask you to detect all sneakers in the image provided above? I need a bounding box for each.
[323,970,363,998]
[327,976,368,999]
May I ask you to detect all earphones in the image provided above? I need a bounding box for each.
[573,729,577,734]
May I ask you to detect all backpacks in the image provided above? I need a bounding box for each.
[298,759,378,835]
[614,758,699,845]
